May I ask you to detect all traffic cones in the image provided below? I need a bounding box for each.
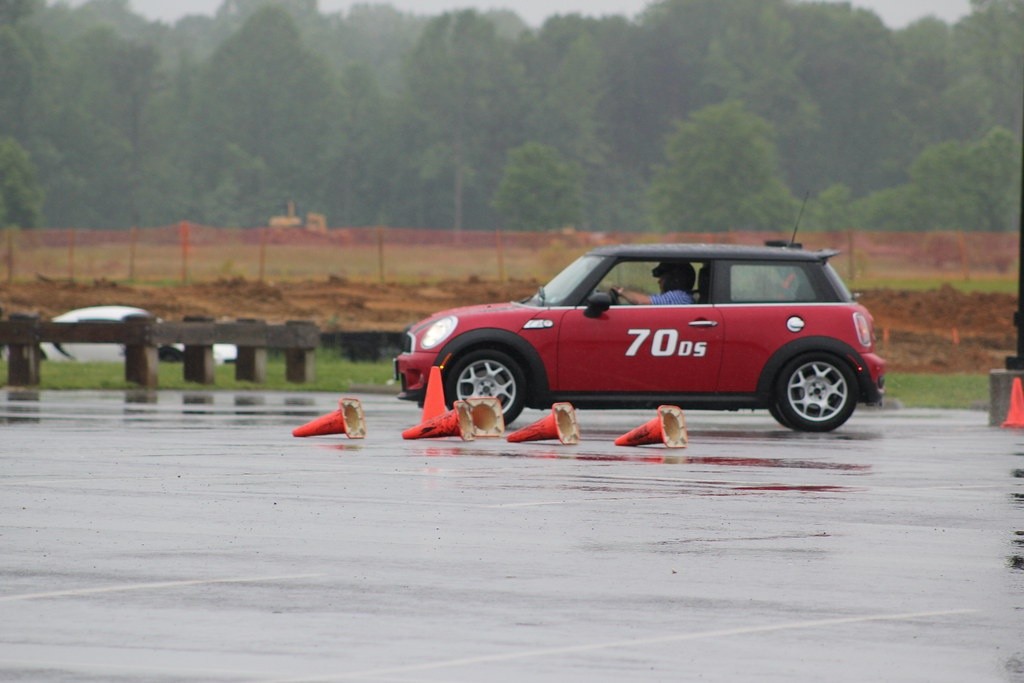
[402,398,477,443]
[504,401,581,448]
[463,396,505,438]
[293,396,367,440]
[613,403,689,450]
[881,326,889,343]
[1000,376,1024,429]
[420,366,447,424]
[951,327,962,347]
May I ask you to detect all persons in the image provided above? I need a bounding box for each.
[611,259,696,305]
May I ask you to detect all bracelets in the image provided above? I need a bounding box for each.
[619,287,624,294]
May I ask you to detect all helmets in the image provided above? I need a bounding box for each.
[653,261,695,290]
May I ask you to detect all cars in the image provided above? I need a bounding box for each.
[0,304,238,366]
[393,241,886,434]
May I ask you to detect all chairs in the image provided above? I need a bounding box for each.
[697,268,711,305]
